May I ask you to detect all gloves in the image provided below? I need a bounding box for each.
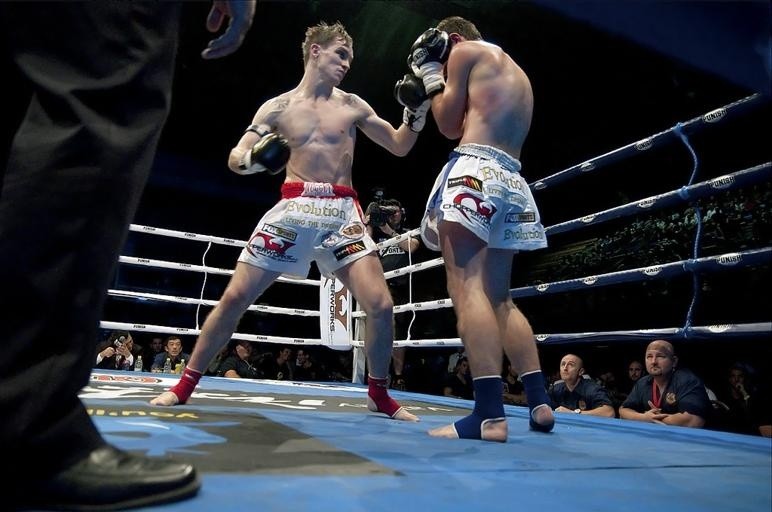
[238,129,291,176]
[406,27,452,97]
[393,72,432,132]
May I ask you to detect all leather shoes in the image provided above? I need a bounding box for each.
[27,439,200,510]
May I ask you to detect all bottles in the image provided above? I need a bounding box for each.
[180,358,186,374]
[162,357,171,374]
[134,355,143,372]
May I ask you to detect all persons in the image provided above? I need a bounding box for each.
[149,20,431,422]
[596,340,770,436]
[444,347,527,405]
[0,0,256,512]
[547,354,616,418]
[91,330,353,383]
[525,183,772,294]
[364,199,421,391]
[407,16,554,443]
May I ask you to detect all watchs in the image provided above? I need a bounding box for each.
[575,409,581,414]
